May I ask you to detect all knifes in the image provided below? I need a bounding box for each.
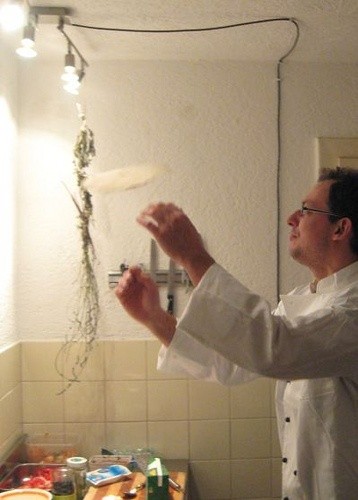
[167,258,175,316]
[149,239,159,283]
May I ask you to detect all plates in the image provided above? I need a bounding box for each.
[0,488,52,500]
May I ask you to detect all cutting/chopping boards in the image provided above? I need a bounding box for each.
[82,471,187,500]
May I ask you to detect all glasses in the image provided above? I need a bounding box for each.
[298,200,343,218]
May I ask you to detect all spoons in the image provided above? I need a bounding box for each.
[123,480,146,496]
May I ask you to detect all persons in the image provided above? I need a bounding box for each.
[115,167,357,500]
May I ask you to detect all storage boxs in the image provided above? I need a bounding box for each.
[0,463,67,492]
